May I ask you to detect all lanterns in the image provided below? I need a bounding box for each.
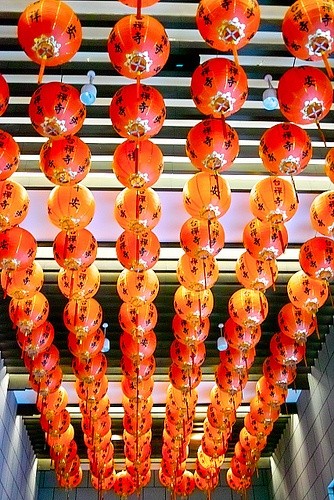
[158,0,334,500]
[108,0,170,500]
[0,0,116,495]
